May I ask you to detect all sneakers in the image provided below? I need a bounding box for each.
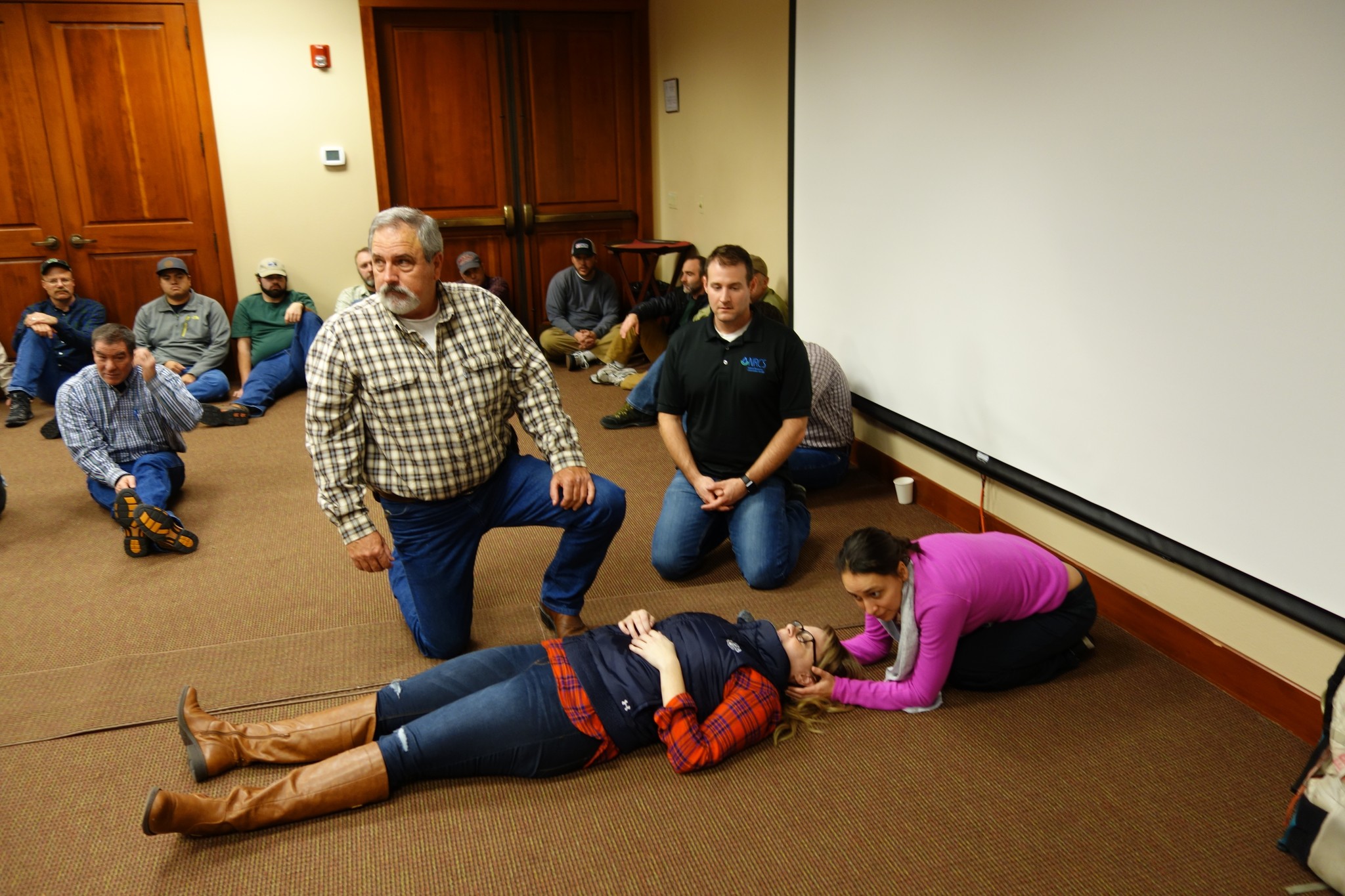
[597,368,637,386]
[198,403,248,427]
[589,362,625,384]
[566,350,590,370]
[4,395,34,423]
[601,402,654,429]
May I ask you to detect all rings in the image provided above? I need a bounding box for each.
[32,317,36,320]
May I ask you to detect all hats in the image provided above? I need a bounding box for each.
[257,257,287,278]
[749,255,767,276]
[156,257,188,275]
[571,238,597,256]
[456,251,481,274]
[41,258,70,274]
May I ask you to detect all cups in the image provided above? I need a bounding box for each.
[893,476,914,504]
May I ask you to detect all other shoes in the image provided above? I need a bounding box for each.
[115,488,148,558]
[1068,634,1096,662]
[784,484,807,505]
[134,504,198,553]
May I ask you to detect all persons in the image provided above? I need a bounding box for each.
[784,527,1098,716]
[651,244,813,588]
[304,207,630,660]
[141,608,864,841]
[0,251,855,557]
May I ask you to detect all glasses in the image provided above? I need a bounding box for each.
[792,620,816,667]
[41,278,74,284]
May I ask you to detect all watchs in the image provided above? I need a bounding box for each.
[740,473,759,494]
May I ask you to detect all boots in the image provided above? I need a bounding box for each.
[142,741,389,837]
[177,685,377,782]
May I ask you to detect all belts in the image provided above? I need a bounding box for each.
[378,483,480,504]
[813,446,847,452]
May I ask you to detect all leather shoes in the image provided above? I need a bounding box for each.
[539,600,589,639]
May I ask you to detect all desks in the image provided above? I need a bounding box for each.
[601,239,692,328]
